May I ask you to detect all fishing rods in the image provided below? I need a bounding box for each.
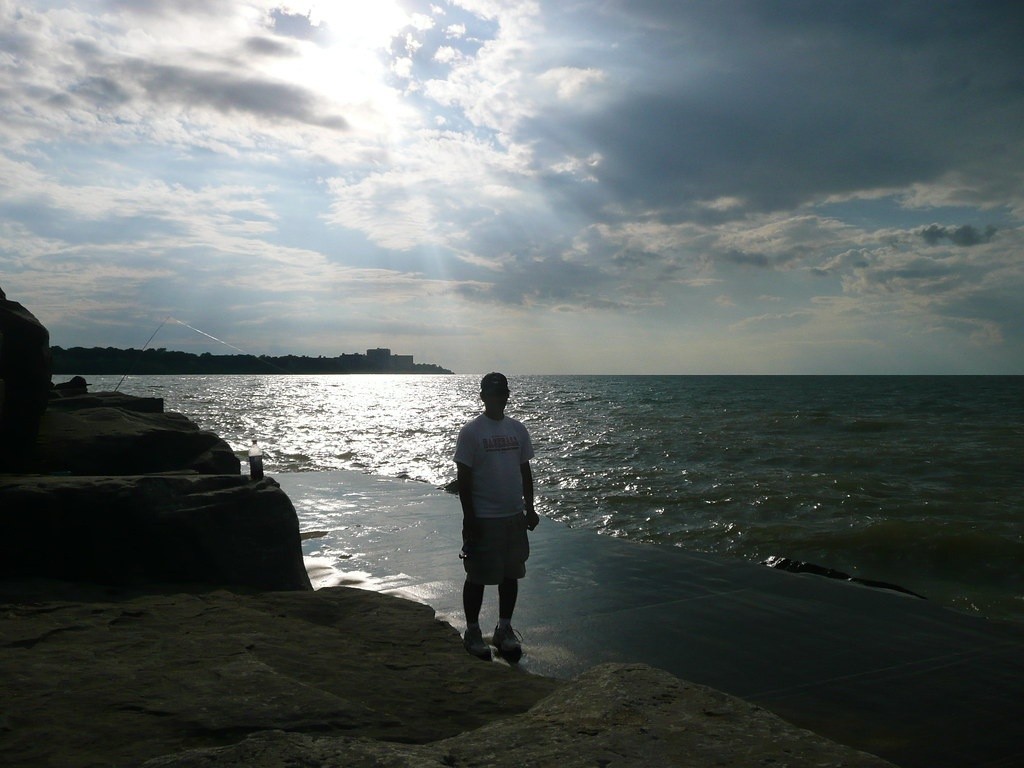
[113,315,171,392]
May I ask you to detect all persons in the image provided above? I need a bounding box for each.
[454,372,539,663]
[67,376,92,393]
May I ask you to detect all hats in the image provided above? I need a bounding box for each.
[480,373,510,394]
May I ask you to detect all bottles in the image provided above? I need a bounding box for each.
[458,539,471,559]
[248,439,263,480]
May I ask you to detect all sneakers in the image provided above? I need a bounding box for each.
[492,625,523,654]
[463,629,491,657]
